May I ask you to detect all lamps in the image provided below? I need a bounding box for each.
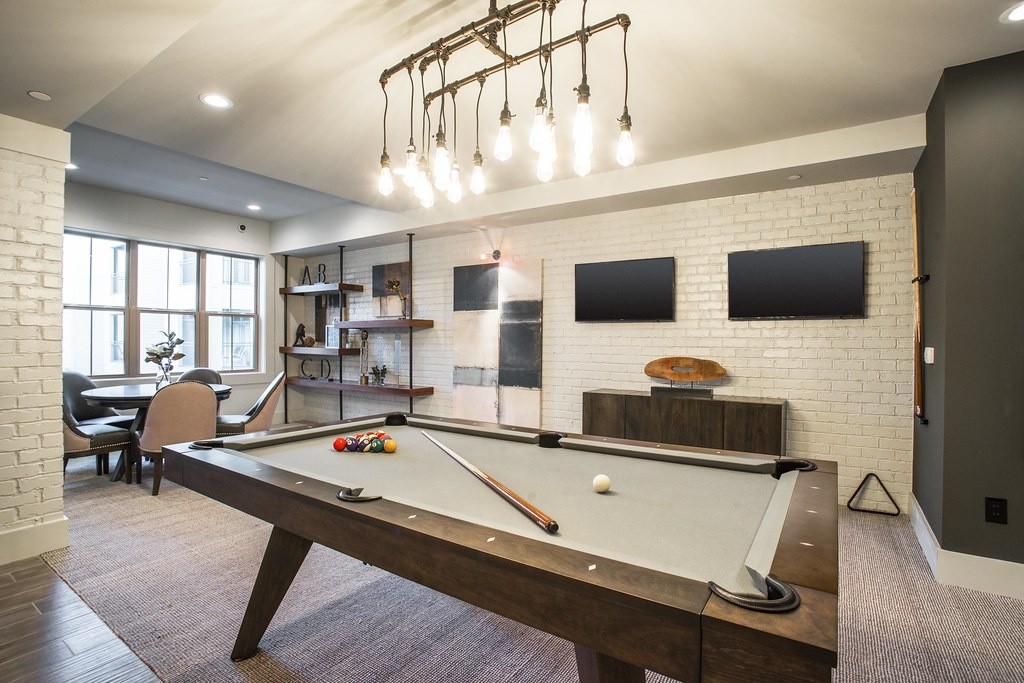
[380,0,636,207]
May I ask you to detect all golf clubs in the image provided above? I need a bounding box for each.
[420,429,559,534]
[910,187,926,417]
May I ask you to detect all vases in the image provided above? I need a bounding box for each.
[155,364,172,390]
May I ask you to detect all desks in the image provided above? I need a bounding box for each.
[81,382,231,481]
[162,407,839,683]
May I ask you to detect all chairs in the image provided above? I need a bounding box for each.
[216,369,285,437]
[62,371,136,474]
[134,380,218,496]
[62,406,133,484]
[177,368,223,415]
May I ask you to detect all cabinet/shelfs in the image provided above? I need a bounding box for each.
[583,388,787,457]
[279,232,433,423]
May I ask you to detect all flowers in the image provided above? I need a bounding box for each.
[145,329,185,383]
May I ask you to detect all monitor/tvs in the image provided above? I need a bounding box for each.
[453,263,500,311]
[575,257,674,321]
[726,241,866,319]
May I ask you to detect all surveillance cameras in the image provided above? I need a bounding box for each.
[238,224,247,232]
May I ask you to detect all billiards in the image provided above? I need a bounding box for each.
[593,474,611,493]
[333,431,397,454]
